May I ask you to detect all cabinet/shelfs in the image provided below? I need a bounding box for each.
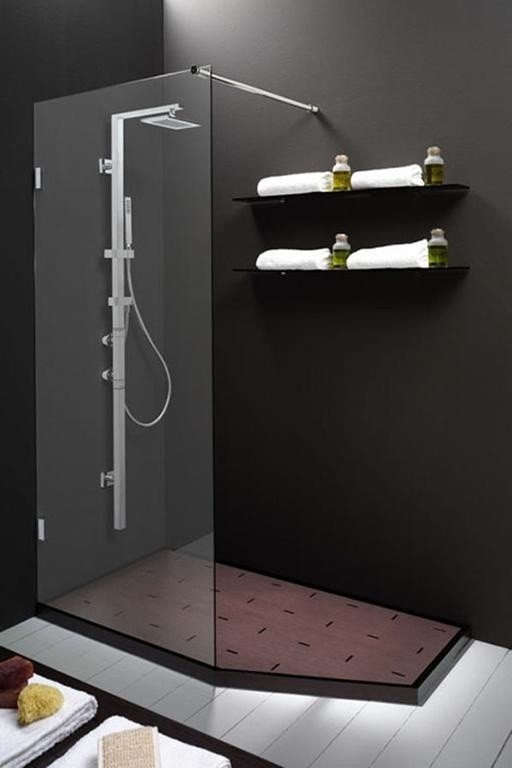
[230,184,469,275]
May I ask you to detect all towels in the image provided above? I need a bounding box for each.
[253,237,429,270]
[0,671,231,767]
[257,163,425,198]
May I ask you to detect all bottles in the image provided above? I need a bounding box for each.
[427,227,450,267]
[424,146,445,185]
[331,234,351,269]
[332,153,350,190]
